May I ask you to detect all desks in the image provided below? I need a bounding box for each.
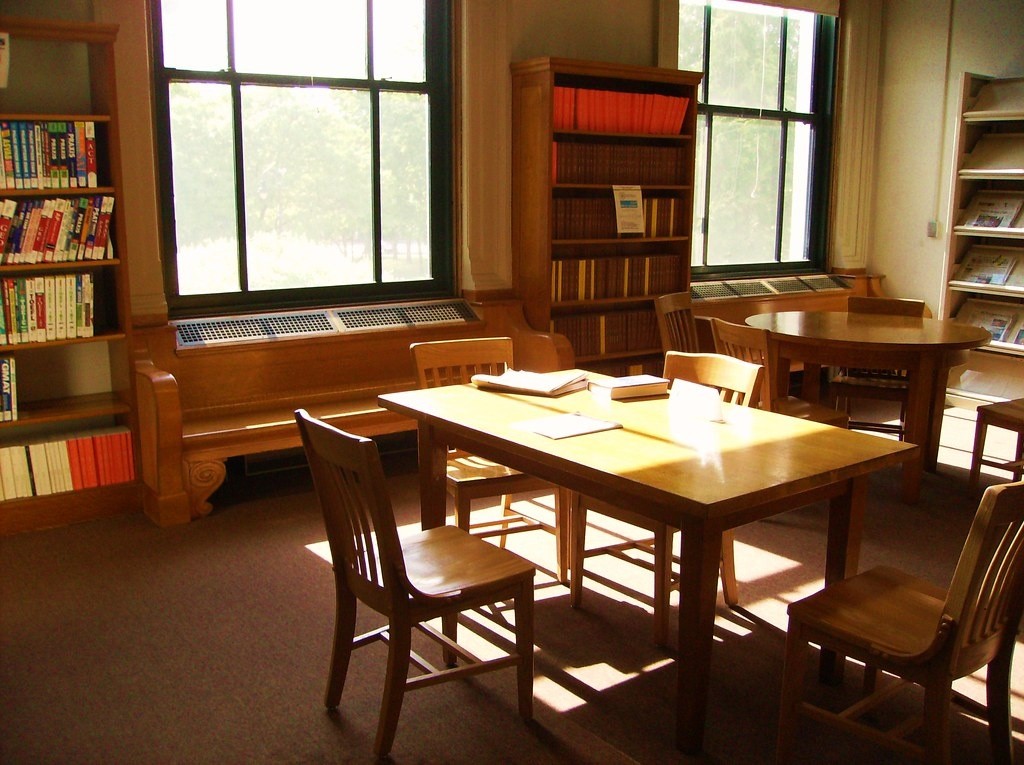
[743,311,991,505]
[377,369,924,756]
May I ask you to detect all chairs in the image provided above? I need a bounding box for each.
[776,477,1023,765]
[409,337,566,584]
[294,408,537,758]
[967,397,1024,485]
[829,294,925,442]
[710,315,848,430]
[653,288,701,358]
[568,349,766,646]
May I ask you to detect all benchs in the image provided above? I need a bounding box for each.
[128,298,575,527]
[690,268,932,374]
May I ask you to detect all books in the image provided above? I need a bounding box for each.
[0,121,96,190]
[0,424,133,504]
[552,139,692,186]
[471,368,591,396]
[0,196,117,267]
[553,83,690,135]
[552,195,684,239]
[0,270,95,346]
[586,373,670,401]
[0,351,17,424]
[578,359,666,381]
[549,251,682,301]
[551,309,673,358]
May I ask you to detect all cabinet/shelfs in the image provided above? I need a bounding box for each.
[0,18,146,536]
[511,56,708,377]
[936,69,1024,412]
